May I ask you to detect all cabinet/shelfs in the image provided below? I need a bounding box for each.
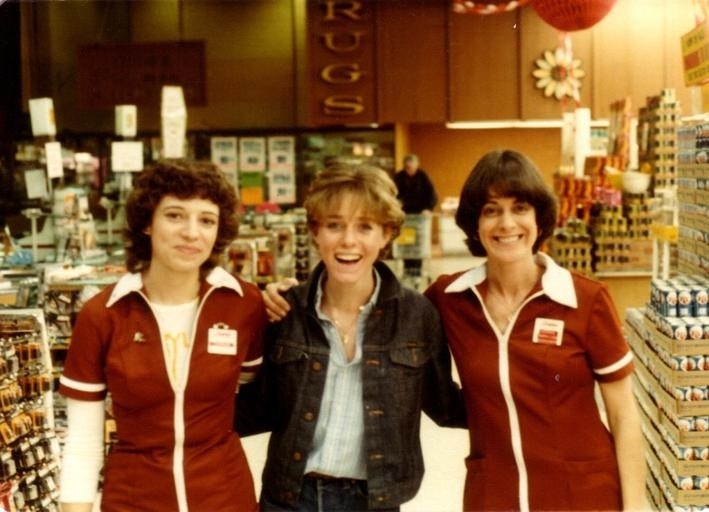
[1,204,398,291]
[546,90,676,283]
[622,113,707,509]
[2,271,119,510]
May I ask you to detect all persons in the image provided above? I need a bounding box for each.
[392,154,437,215]
[57,157,271,512]
[233,163,469,512]
[262,149,652,512]
